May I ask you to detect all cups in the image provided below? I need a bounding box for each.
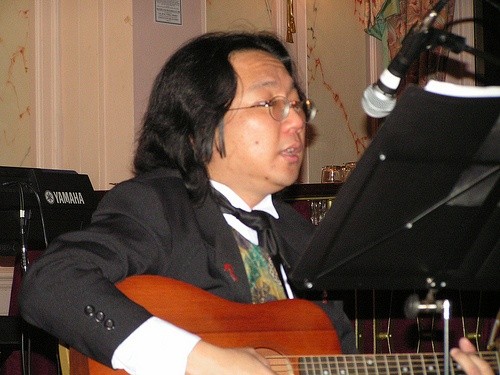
[321,162,357,182]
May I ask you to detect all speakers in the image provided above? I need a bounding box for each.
[0,166,96,254]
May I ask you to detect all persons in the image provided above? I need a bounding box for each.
[18,32,494,375]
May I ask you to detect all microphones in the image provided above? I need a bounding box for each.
[362,8,439,118]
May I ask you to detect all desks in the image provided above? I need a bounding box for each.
[281,182,344,225]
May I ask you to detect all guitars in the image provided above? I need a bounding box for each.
[70,273,500,375]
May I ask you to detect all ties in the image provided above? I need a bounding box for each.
[211,187,290,299]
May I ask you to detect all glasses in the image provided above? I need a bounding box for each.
[228,96,319,123]
